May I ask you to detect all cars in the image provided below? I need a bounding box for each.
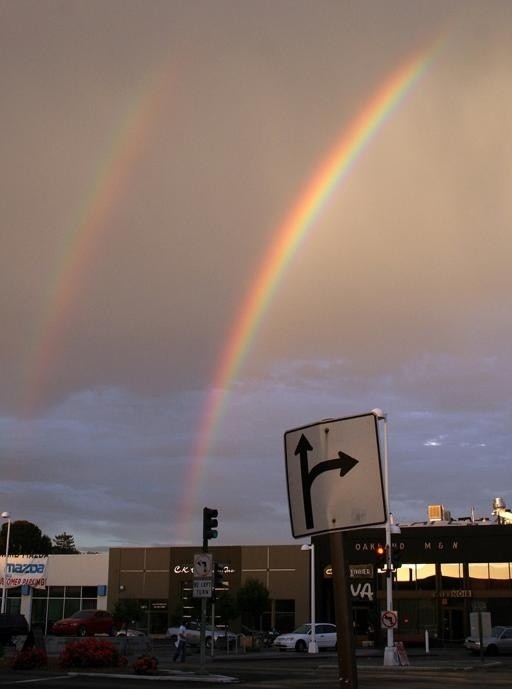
[464,625,512,657]
[167,621,238,649]
[273,623,337,653]
[52,610,145,636]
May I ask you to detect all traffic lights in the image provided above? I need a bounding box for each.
[203,507,218,539]
[376,546,385,568]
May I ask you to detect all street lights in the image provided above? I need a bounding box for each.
[0,511,11,614]
[372,407,400,666]
[301,543,318,653]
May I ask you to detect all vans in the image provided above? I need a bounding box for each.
[0,614,29,645]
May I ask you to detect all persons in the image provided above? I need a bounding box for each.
[172,618,189,663]
